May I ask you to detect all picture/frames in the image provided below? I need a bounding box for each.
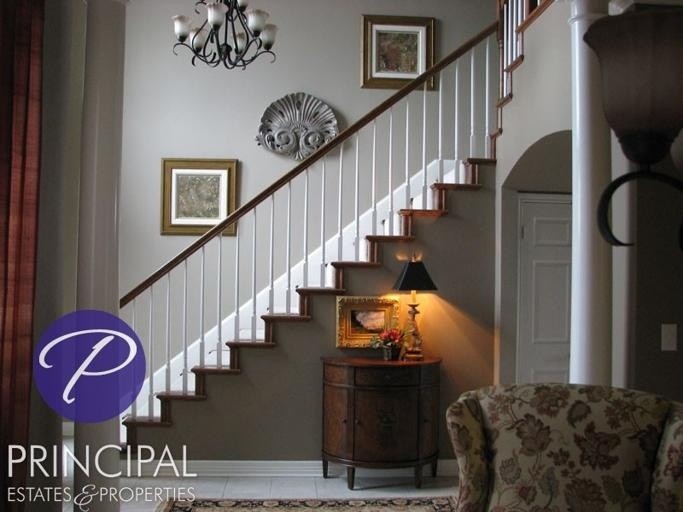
[335,294,400,350]
[359,13,437,92]
[159,157,238,237]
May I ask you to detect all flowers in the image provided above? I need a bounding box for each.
[369,318,409,350]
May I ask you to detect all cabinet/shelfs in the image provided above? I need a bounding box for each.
[320,356,442,490]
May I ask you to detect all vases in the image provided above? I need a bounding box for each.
[384,348,402,360]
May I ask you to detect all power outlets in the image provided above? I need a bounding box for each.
[661,323,678,352]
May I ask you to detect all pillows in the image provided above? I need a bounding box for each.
[477,381,671,512]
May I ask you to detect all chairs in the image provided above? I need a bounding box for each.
[444,391,683,512]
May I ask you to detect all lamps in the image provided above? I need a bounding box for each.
[391,251,438,361]
[171,1,278,71]
[582,1,683,248]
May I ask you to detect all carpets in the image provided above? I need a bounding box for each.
[153,494,458,512]
